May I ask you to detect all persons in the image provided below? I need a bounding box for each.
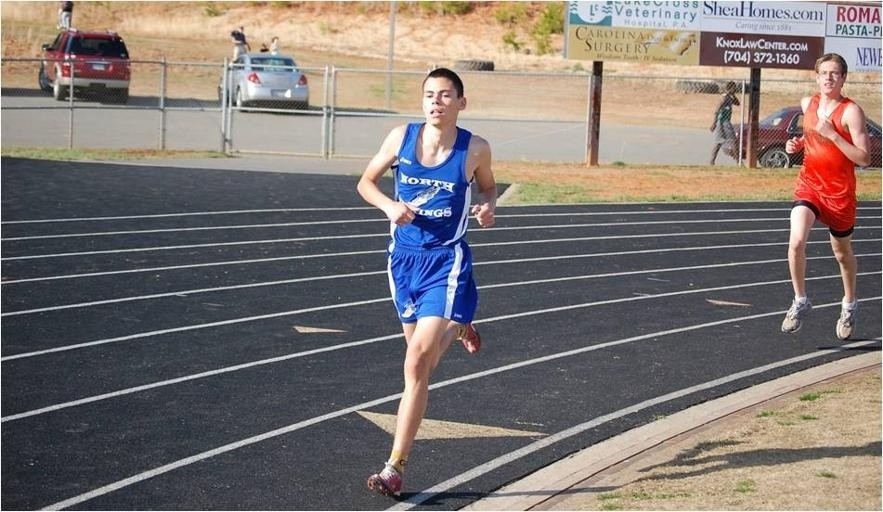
[272,34,280,55]
[355,67,498,494]
[58,1,73,30]
[231,28,250,60]
[260,44,269,52]
[782,52,870,341]
[708,82,744,165]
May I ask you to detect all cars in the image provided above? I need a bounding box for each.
[723,106,882,168]
[219,53,308,112]
[40,28,130,105]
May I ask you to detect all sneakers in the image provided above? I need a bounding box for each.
[367,464,403,499]
[781,298,814,335]
[835,296,858,341]
[459,318,482,353]
